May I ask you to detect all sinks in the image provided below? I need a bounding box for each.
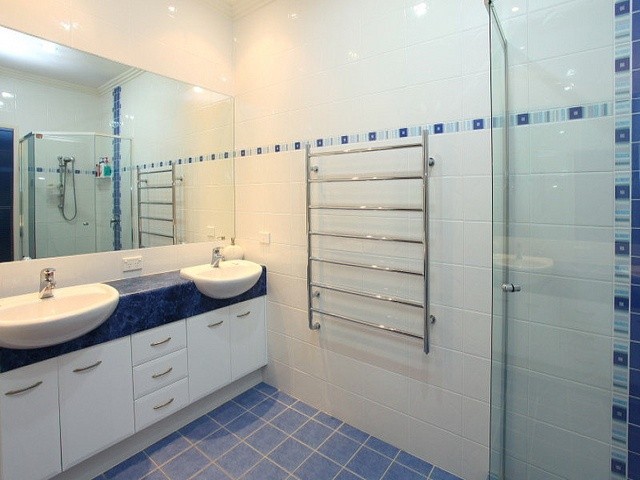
[180,260,263,298]
[1,283,119,349]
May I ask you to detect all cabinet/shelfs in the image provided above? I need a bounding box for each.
[186,306,230,404]
[228,295,268,382]
[130,319,188,433]
[136,161,183,249]
[57,335,136,471]
[305,129,435,355]
[0,357,62,480]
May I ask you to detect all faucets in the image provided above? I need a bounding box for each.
[210,246,228,269]
[38,268,60,296]
